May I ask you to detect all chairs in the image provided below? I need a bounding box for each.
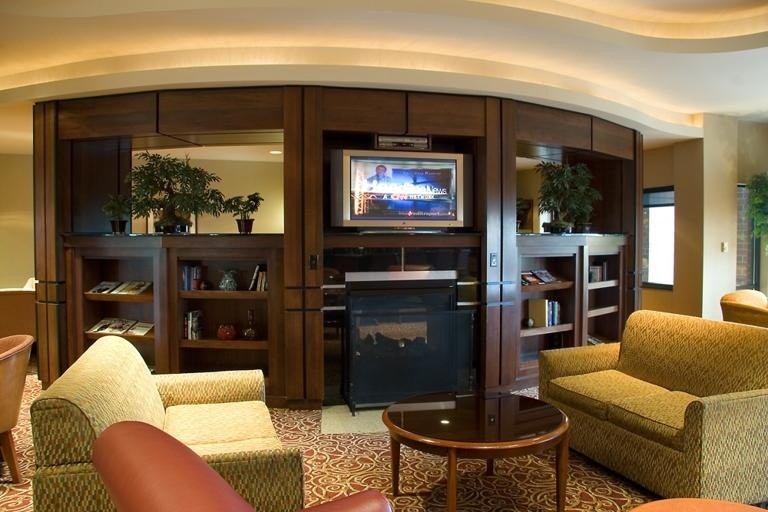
[0,334,34,485]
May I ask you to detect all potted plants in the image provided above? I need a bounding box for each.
[534,160,592,234]
[102,195,132,234]
[575,187,602,233]
[122,150,225,235]
[223,192,264,234]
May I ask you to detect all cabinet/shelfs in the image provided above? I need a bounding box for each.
[302,85,500,412]
[501,97,642,394]
[33,84,304,410]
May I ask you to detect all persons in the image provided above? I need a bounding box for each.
[367,164,392,184]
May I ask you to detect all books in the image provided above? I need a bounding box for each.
[521,267,556,286]
[111,280,152,296]
[589,261,610,283]
[181,265,203,292]
[87,317,154,338]
[183,309,201,342]
[248,263,266,293]
[87,280,122,294]
[527,297,561,328]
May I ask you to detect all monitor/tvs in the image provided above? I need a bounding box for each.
[331,148,474,234]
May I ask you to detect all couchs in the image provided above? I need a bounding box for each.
[538,310,768,505]
[720,290,768,329]
[93,422,390,512]
[31,335,304,512]
